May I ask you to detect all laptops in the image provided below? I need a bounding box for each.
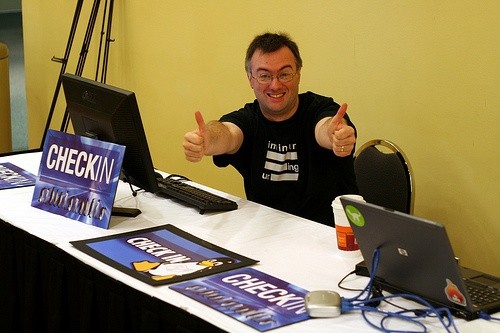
[339,196,500,315]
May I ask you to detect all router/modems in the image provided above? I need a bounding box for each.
[306,290,342,318]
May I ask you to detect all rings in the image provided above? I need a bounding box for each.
[341,146,345,151]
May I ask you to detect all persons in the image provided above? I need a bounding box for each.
[183,33,357,229]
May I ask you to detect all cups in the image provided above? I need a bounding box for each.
[331,195,366,251]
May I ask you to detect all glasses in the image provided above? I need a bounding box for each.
[251,69,298,83]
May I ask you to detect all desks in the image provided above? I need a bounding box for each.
[0,150,500,333]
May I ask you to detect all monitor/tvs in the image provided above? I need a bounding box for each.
[60,73,159,194]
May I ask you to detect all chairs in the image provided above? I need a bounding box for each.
[353,140,414,217]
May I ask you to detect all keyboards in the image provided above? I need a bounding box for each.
[152,175,238,214]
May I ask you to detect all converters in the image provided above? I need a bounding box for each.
[355,261,372,277]
